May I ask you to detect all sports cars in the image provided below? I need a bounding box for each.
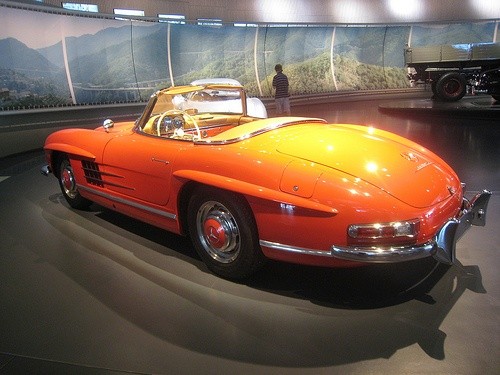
[172,78,268,118]
[41,84,492,279]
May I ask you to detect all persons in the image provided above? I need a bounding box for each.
[272,63,291,118]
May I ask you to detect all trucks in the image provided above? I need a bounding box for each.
[404,40,498,103]
